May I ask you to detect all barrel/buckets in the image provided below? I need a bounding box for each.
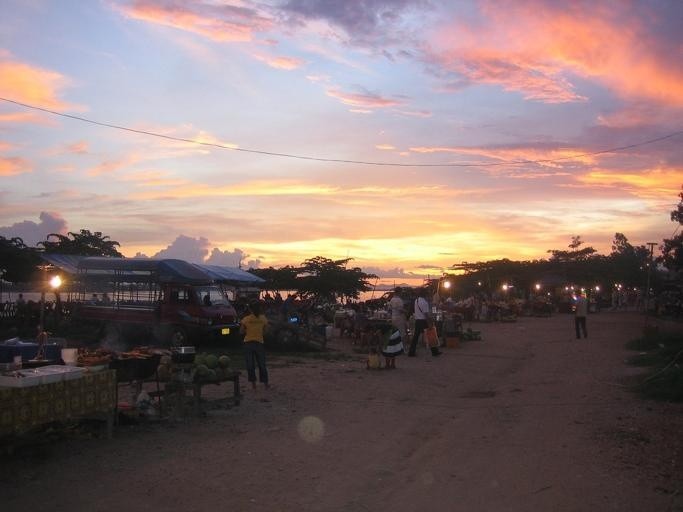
[445,336,460,348]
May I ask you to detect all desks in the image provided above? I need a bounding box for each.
[0,369,117,441]
[164,371,243,415]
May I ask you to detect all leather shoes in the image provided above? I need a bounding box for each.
[433,352,442,355]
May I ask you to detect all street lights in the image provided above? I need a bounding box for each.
[32,260,62,360]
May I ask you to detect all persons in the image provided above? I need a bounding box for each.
[449,284,682,317]
[18,284,442,390]
[573,287,588,338]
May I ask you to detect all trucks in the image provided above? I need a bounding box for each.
[75,256,241,349]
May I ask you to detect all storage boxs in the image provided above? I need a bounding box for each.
[0,343,61,362]
